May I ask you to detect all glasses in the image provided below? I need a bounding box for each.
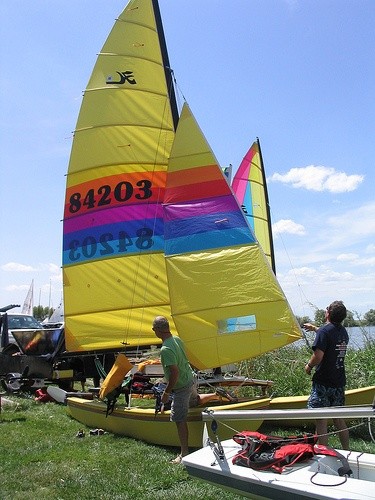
[326,307,329,311]
[152,327,160,331]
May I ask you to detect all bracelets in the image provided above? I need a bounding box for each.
[163,390,170,395]
[308,363,313,369]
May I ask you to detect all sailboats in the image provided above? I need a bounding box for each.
[162,100,375,427]
[62,0,272,448]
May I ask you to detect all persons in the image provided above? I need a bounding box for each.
[302,300,349,450]
[153,315,237,464]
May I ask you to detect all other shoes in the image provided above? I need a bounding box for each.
[169,455,183,464]
[216,388,238,402]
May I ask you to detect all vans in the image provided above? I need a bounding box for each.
[0,314,44,343]
